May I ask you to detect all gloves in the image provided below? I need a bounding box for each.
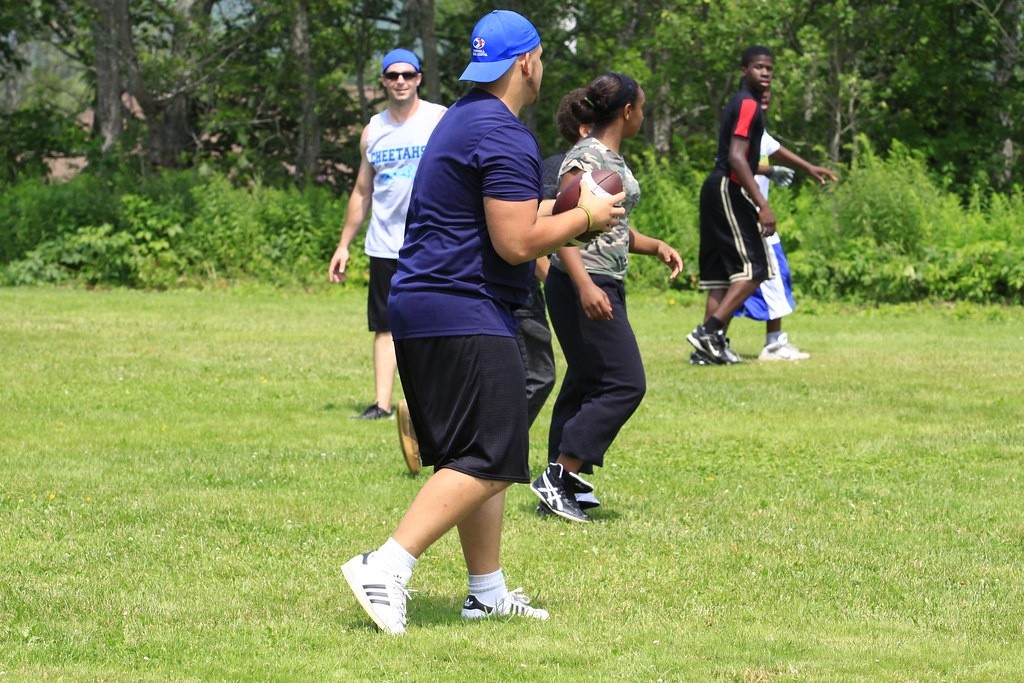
[767,166,796,187]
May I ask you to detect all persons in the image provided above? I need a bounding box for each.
[531,72,683,526]
[333,8,633,645]
[745,77,839,362]
[328,46,454,422]
[687,45,796,364]
[387,82,610,473]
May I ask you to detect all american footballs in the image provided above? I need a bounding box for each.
[552,169,622,247]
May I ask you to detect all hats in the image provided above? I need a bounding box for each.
[457,9,541,83]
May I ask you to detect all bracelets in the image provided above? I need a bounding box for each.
[577,201,596,233]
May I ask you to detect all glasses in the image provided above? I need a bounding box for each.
[384,71,417,80]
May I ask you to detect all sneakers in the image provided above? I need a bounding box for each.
[758,331,810,360]
[351,403,395,420]
[459,587,549,620]
[396,400,420,475]
[340,549,418,634]
[530,462,600,523]
[686,324,741,366]
